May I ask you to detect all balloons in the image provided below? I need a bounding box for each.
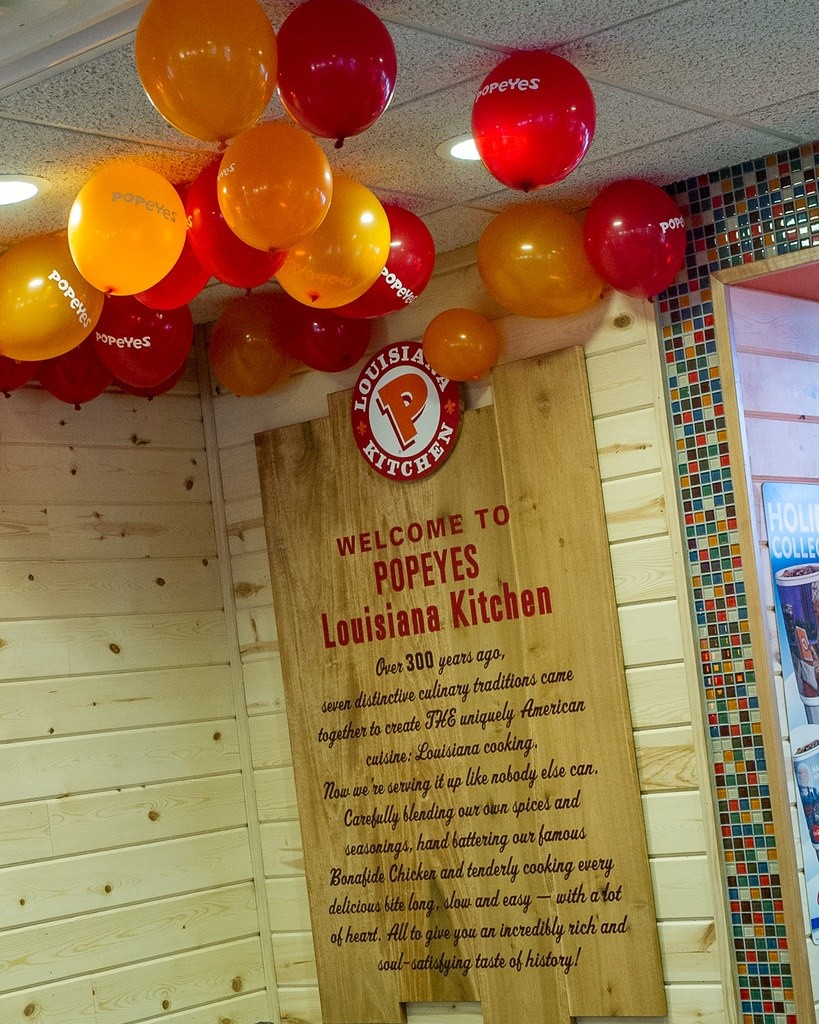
[272,287,371,373]
[219,120,333,252]
[331,205,434,319]
[210,294,284,397]
[277,176,391,307]
[67,161,186,296]
[92,296,193,398]
[0,236,105,361]
[472,51,597,191]
[422,308,499,382]
[477,203,605,317]
[276,1,399,148]
[185,155,290,289]
[135,1,279,151]
[0,336,113,403]
[586,179,687,302]
[132,180,210,311]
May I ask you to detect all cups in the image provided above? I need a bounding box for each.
[775,563,819,707]
[792,738,819,851]
